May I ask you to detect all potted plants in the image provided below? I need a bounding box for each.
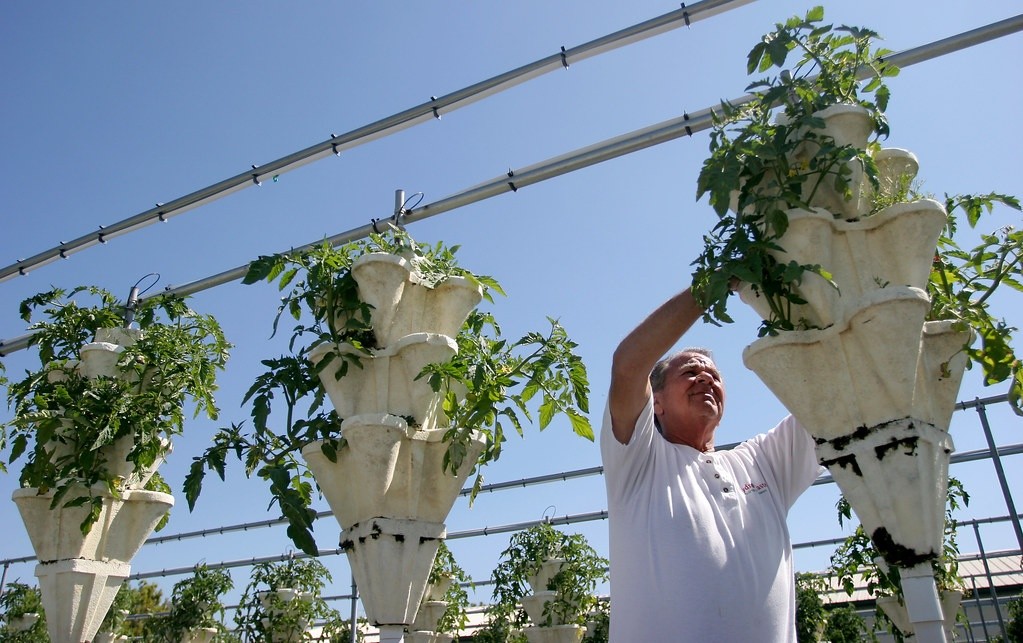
[0,6,1022,640]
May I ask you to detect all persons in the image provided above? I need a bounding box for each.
[600,264,828,643]
